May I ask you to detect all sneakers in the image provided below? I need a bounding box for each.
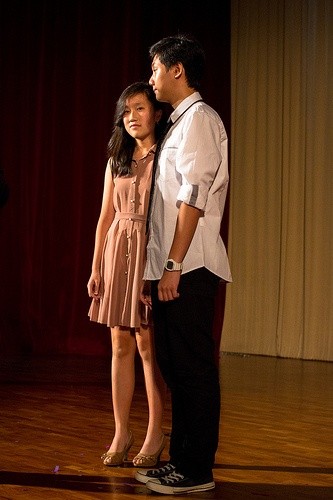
[136,462,176,483]
[146,470,215,494]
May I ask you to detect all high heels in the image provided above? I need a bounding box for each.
[101,426,135,466]
[133,433,166,466]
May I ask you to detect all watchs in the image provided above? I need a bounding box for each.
[163,260,182,272]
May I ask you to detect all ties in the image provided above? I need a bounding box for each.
[145,100,204,235]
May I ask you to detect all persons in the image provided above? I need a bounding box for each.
[87,81,165,467]
[135,38,232,493]
